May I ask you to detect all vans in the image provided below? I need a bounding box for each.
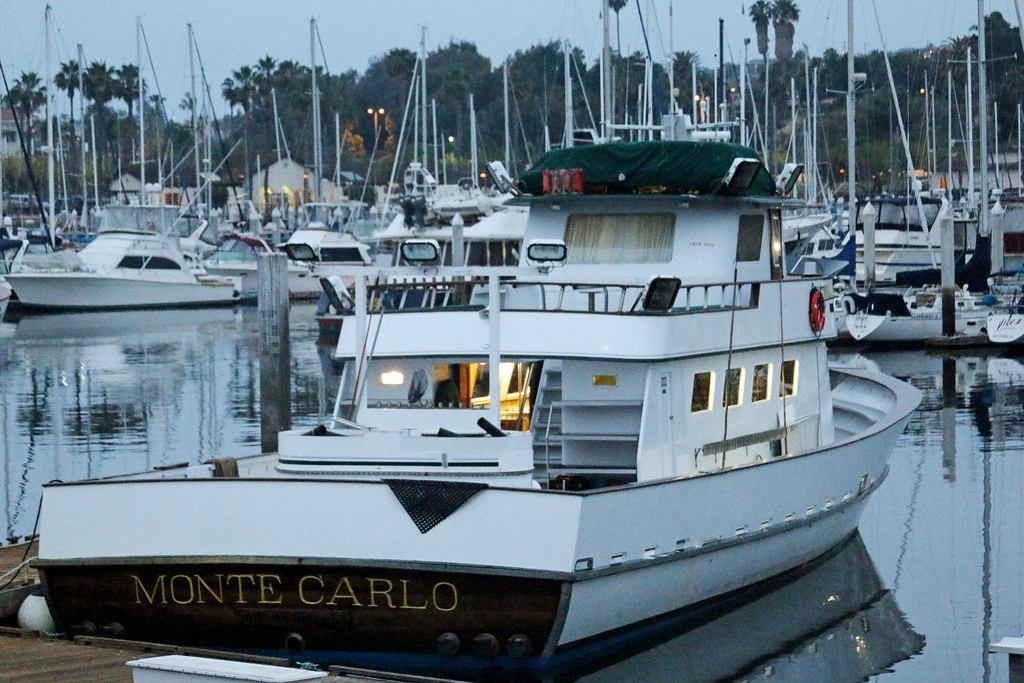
[9,193,41,210]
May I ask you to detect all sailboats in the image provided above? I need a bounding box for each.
[0,0,1023,347]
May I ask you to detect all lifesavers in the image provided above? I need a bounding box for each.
[841,295,856,314]
[811,290,825,331]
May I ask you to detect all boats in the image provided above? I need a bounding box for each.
[5,199,240,310]
[480,526,926,683]
[13,305,242,374]
[31,144,926,674]
[1,535,44,620]
